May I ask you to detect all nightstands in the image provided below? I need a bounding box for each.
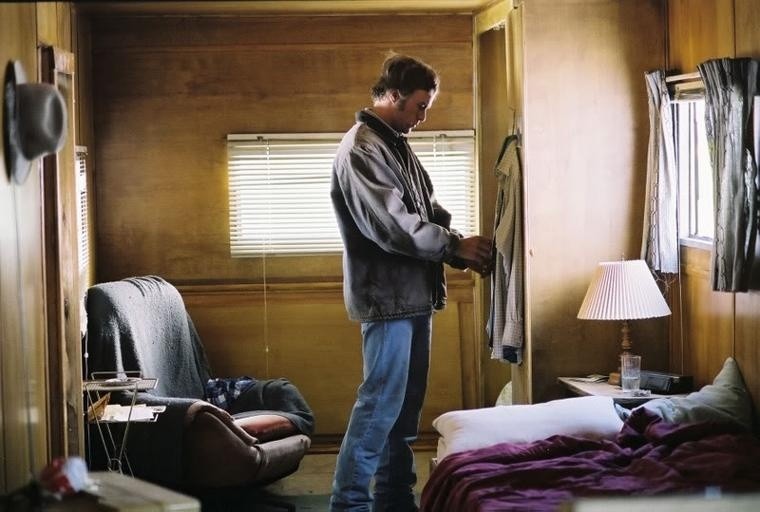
[556,376,688,410]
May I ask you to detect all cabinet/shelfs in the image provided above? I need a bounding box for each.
[81,371,168,480]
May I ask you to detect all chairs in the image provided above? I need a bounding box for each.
[84,274,315,489]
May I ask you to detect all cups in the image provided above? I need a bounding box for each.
[620,355,641,394]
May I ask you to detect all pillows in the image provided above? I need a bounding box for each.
[631,357,759,426]
[433,395,625,463]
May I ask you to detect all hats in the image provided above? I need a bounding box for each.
[3,57,70,184]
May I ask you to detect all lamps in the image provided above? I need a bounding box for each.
[576,253,673,385]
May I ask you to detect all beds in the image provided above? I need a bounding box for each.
[419,407,758,512]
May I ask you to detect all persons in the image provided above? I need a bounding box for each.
[328,54,494,511]
